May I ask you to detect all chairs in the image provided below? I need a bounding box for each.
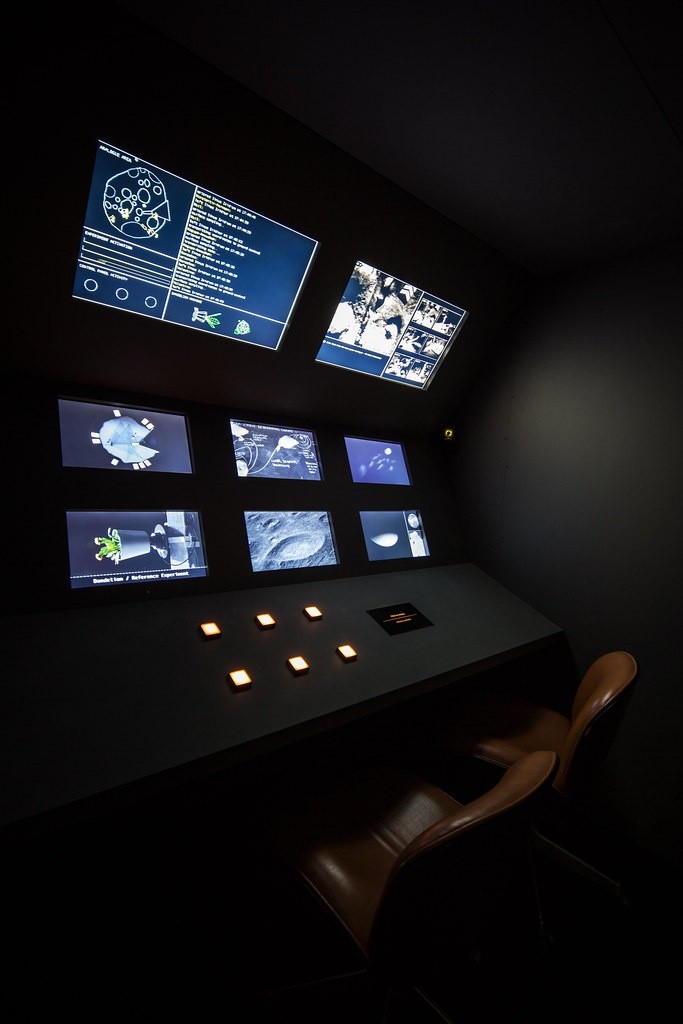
[259,749,560,1024]
[426,649,642,879]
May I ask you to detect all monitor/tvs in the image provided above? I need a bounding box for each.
[359,510,430,561]
[314,257,469,391]
[73,139,319,353]
[344,436,412,486]
[230,419,323,481]
[244,511,337,572]
[58,397,195,474]
[66,511,208,588]
